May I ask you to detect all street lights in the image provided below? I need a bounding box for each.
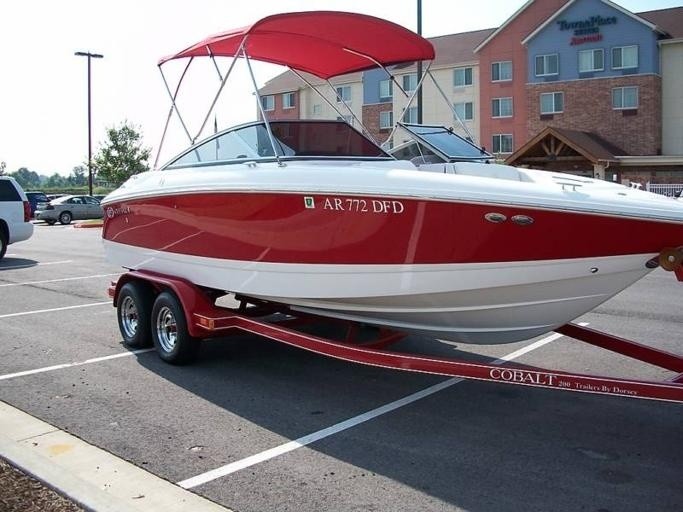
[74,52,104,194]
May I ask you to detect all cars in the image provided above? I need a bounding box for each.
[0,176,104,259]
